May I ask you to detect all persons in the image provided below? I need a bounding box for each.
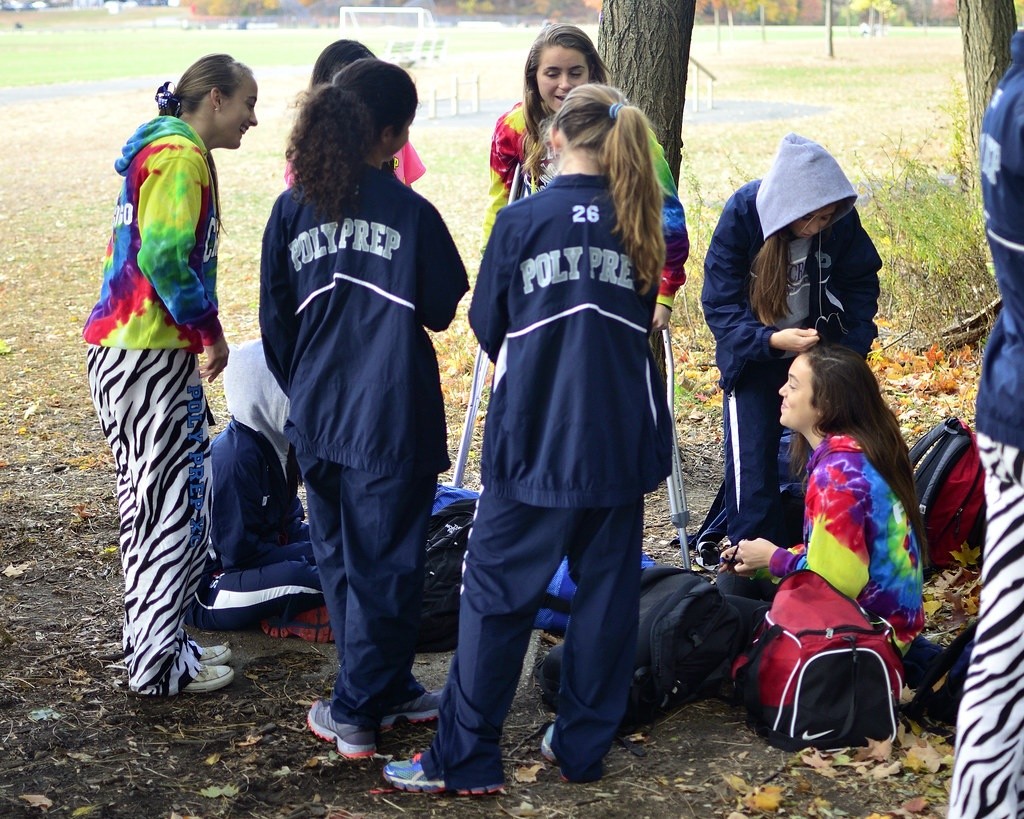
[949,27,1023,819]
[708,341,926,708]
[478,22,692,333]
[185,336,327,632]
[80,53,238,696]
[284,40,426,189]
[256,59,470,762]
[699,132,883,550]
[381,87,674,798]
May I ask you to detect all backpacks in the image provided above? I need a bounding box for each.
[424,496,481,650]
[535,565,737,736]
[908,417,986,570]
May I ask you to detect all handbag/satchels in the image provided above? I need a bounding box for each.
[432,482,655,633]
[733,569,905,755]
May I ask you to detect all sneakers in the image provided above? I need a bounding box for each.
[540,724,571,785]
[261,607,336,644]
[198,645,233,666]
[383,754,506,796]
[306,698,376,759]
[381,690,441,733]
[182,663,235,692]
